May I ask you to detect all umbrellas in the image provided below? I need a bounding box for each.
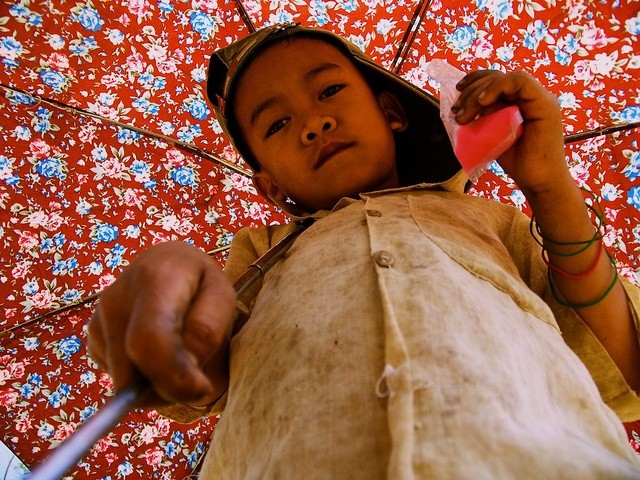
[0,1,639,480]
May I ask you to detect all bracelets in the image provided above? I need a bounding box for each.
[529,186,617,307]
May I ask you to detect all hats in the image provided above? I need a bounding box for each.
[205,24,473,193]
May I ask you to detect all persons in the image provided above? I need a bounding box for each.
[87,22,640,480]
